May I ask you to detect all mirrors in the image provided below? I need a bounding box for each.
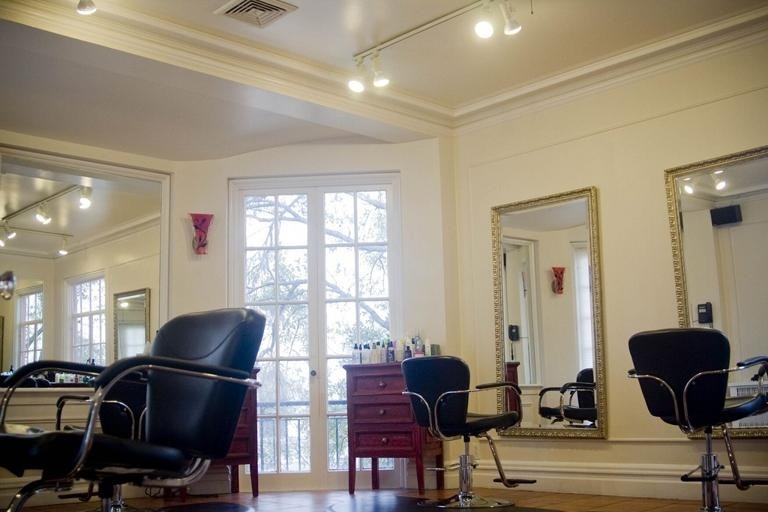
[490,186,607,440]
[0,138,173,391]
[113,287,151,362]
[663,144,768,440]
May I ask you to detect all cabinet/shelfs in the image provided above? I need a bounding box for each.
[342,364,444,494]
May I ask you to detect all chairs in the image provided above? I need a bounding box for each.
[398,355,535,508]
[625,329,768,511]
[538,368,598,428]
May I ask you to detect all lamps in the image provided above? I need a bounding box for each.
[76,1,97,15]
[684,173,726,194]
[348,1,522,93]
[0,185,93,256]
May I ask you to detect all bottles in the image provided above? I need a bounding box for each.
[344,334,439,362]
[54,357,95,385]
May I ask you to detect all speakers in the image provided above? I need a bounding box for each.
[710,205,743,226]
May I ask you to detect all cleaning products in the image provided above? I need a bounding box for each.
[48,360,97,383]
[352,328,431,365]
